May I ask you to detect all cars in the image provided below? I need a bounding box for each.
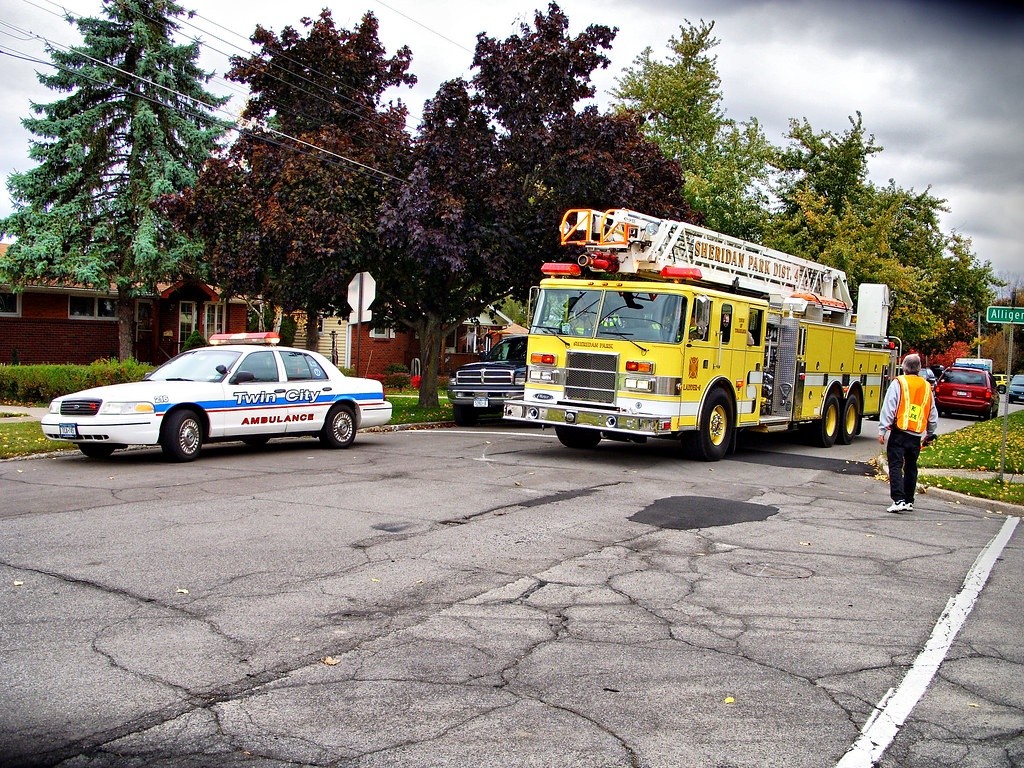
[896,365,938,387]
[1008,374,1024,403]
[41,331,392,462]
[931,366,1006,421]
[991,373,1014,394]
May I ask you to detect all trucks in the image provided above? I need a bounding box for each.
[955,358,993,377]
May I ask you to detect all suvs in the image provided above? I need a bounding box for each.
[447,332,532,428]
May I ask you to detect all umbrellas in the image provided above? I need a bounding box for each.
[489,324,529,335]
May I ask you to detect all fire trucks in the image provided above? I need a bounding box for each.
[502,207,902,462]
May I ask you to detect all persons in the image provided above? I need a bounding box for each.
[569,306,754,346]
[878,354,938,513]
[476,340,487,362]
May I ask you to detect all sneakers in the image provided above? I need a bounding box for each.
[906,502,914,511]
[886,499,906,513]
